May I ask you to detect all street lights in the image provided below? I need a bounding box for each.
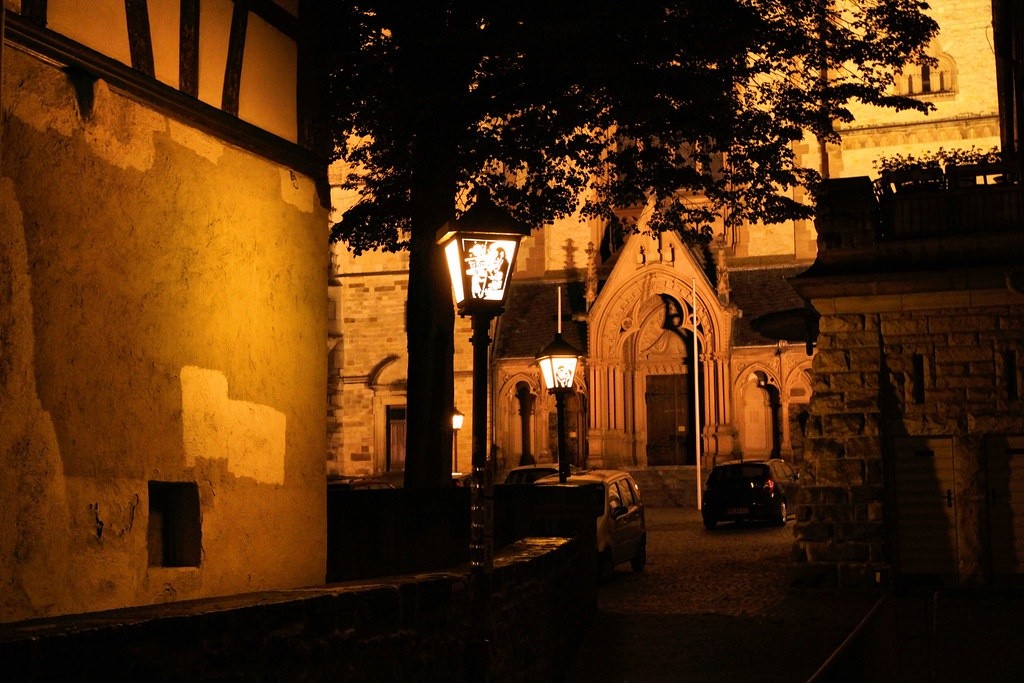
[434,184,538,683]
[535,331,584,485]
[451,406,465,474]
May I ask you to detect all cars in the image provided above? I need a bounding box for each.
[504,464,583,488]
[701,455,796,530]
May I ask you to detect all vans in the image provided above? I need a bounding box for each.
[530,463,647,579]
